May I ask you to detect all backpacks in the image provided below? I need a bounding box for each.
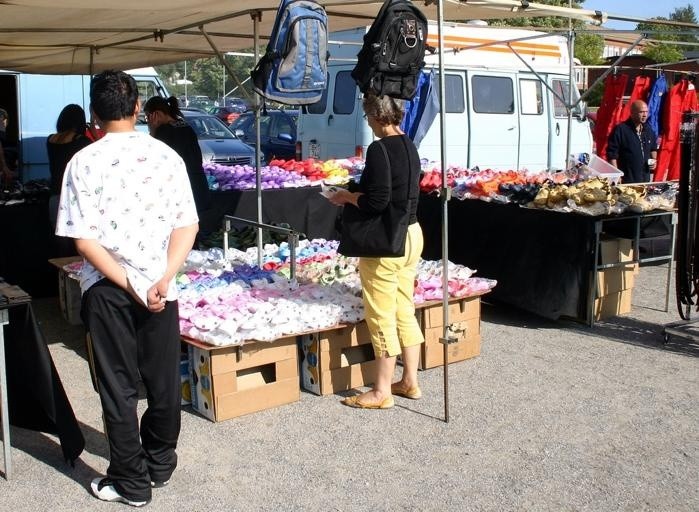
[351,0,436,100]
[251,0,330,115]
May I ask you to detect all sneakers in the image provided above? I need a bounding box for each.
[90,478,169,507]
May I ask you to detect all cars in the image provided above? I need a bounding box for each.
[175,92,250,126]
[177,105,268,168]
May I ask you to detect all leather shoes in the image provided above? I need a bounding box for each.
[1,180,51,205]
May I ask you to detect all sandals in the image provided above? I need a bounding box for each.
[201,157,368,192]
[415,258,496,302]
[176,237,365,347]
[341,390,394,409]
[391,382,422,399]
[416,159,677,220]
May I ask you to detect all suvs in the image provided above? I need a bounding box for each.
[227,107,302,161]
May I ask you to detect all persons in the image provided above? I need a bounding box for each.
[143,95,212,250]
[606,99,658,184]
[0,107,16,184]
[178,92,186,107]
[327,93,427,410]
[54,68,202,507]
[47,103,101,257]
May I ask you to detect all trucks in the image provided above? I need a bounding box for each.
[0,66,174,191]
[296,20,597,173]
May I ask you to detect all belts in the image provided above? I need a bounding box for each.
[677,114,699,322]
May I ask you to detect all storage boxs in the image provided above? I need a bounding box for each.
[299,315,398,395]
[181,335,302,424]
[396,296,480,372]
[592,237,639,322]
[567,151,625,186]
[59,269,82,323]
